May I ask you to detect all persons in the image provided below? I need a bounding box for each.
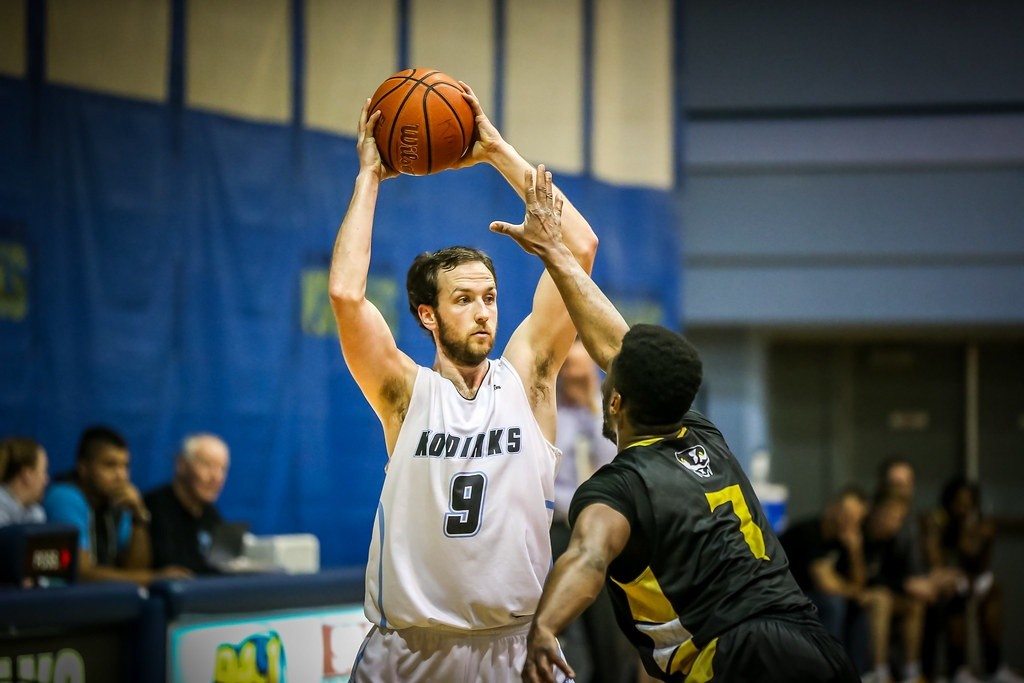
[486,163,865,683]
[544,333,642,683]
[776,458,1024,683]
[1,424,249,588]
[325,79,602,683]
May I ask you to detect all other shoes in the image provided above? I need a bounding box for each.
[992,666,1024,683]
[955,669,981,683]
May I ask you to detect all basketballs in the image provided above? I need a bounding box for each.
[367,67,476,178]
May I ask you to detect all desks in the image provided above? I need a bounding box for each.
[0,568,374,683]
